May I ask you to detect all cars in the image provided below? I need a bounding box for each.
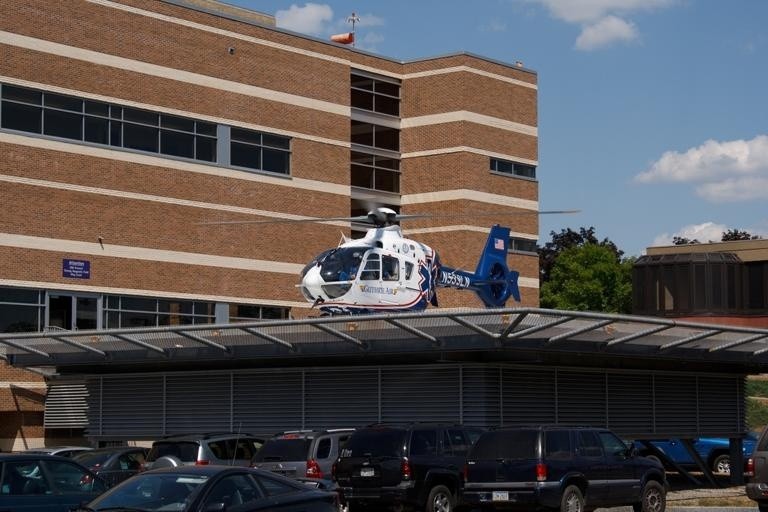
[634,433,761,484]
[331,421,492,511]
[746,423,767,511]
[462,425,669,512]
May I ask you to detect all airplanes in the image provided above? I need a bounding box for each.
[193,205,582,315]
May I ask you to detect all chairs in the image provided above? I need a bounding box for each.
[414,437,431,454]
[3,472,38,494]
[162,479,242,507]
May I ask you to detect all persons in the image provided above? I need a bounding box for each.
[338,252,369,281]
